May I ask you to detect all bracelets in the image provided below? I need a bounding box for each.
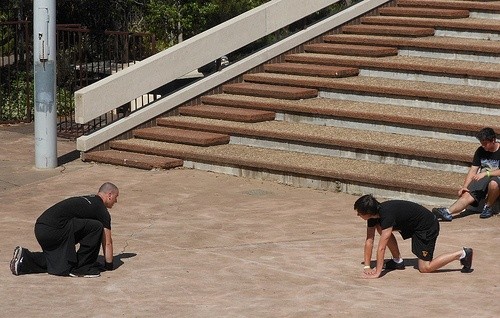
[105,260,113,269]
[363,266,370,269]
[486,170,489,178]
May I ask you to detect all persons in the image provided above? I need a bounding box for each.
[10,182,119,278]
[432,127,500,221]
[354,194,472,278]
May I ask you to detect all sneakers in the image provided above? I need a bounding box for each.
[70,266,101,278]
[480,204,495,219]
[460,247,473,273]
[432,208,452,221]
[382,259,405,270]
[10,246,27,276]
